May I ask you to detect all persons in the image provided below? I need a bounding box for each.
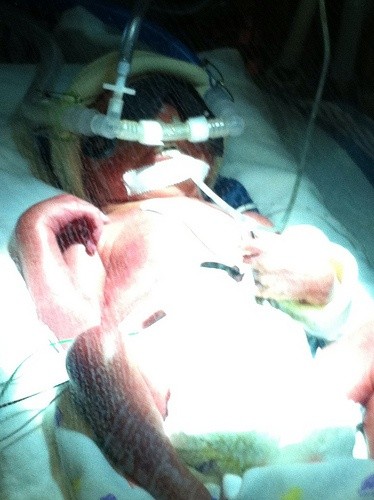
[10,46,374,499]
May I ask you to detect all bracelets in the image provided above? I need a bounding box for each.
[273,241,360,336]
